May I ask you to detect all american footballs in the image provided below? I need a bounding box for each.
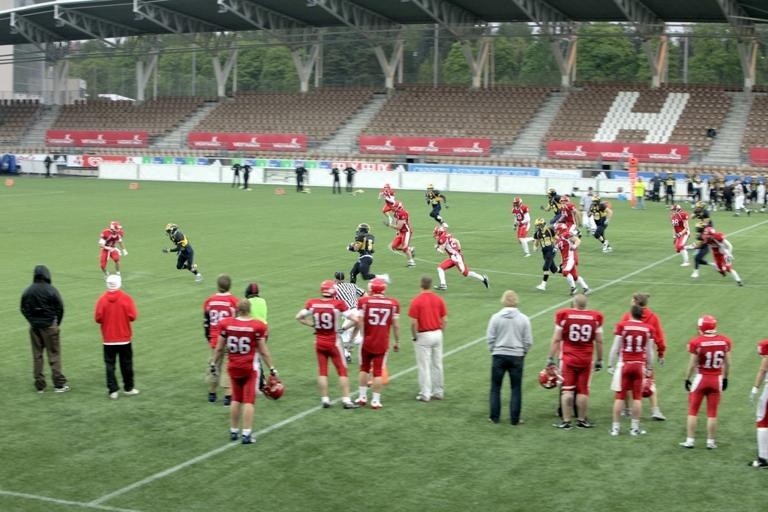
[353,243,360,250]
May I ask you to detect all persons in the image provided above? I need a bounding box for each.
[579,188,599,236]
[689,201,713,238]
[296,280,358,410]
[633,177,646,210]
[670,204,698,267]
[614,294,668,423]
[162,222,203,282]
[97,221,128,279]
[554,300,586,418]
[376,183,402,226]
[407,276,449,402]
[424,184,450,231]
[342,163,358,192]
[706,124,717,139]
[346,224,390,286]
[532,217,564,291]
[230,162,243,188]
[678,313,731,450]
[545,292,604,430]
[511,197,537,257]
[94,273,137,398]
[648,171,664,203]
[684,170,768,216]
[43,155,54,179]
[349,278,401,410]
[685,222,718,278]
[294,163,308,192]
[746,338,768,469]
[205,275,241,403]
[557,196,582,240]
[539,188,563,225]
[210,298,279,442]
[239,165,253,191]
[663,171,677,207]
[245,284,268,393]
[328,165,342,196]
[702,227,743,286]
[554,223,595,296]
[587,195,614,253]
[20,266,69,394]
[384,200,417,270]
[485,288,533,425]
[606,307,654,436]
[432,227,489,291]
[331,273,367,363]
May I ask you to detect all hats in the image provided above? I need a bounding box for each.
[106,274,121,289]
[336,272,343,280]
[501,289,518,307]
[634,293,649,305]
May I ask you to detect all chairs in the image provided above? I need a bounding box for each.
[51,94,220,137]
[0,99,41,142]
[540,80,744,157]
[361,83,563,152]
[191,86,373,140]
[740,85,768,149]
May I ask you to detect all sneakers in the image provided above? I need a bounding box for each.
[482,274,488,289]
[195,273,203,282]
[242,433,255,444]
[433,286,446,290]
[345,402,358,409]
[190,265,196,273]
[54,386,70,392]
[650,409,665,420]
[406,260,416,267]
[576,418,597,426]
[122,389,138,396]
[208,391,216,402]
[737,281,743,287]
[108,389,117,400]
[321,401,332,408]
[229,430,237,440]
[680,262,689,266]
[748,459,767,469]
[705,441,718,449]
[36,387,44,393]
[536,284,545,290]
[415,395,429,402]
[354,397,365,406]
[570,288,576,296]
[690,271,699,278]
[553,420,573,429]
[678,439,695,446]
[582,289,590,295]
[223,394,231,406]
[369,399,382,408]
[628,426,645,436]
[606,426,620,434]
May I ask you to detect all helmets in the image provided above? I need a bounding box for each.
[318,281,336,297]
[355,224,369,235]
[703,227,713,235]
[554,222,566,234]
[264,374,284,402]
[669,204,683,213]
[427,184,434,191]
[696,316,716,334]
[367,278,387,295]
[392,202,403,213]
[512,196,522,205]
[534,217,544,227]
[694,200,708,211]
[558,196,568,204]
[539,366,564,390]
[385,184,390,191]
[164,223,175,234]
[432,225,446,239]
[108,222,120,232]
[590,194,599,207]
